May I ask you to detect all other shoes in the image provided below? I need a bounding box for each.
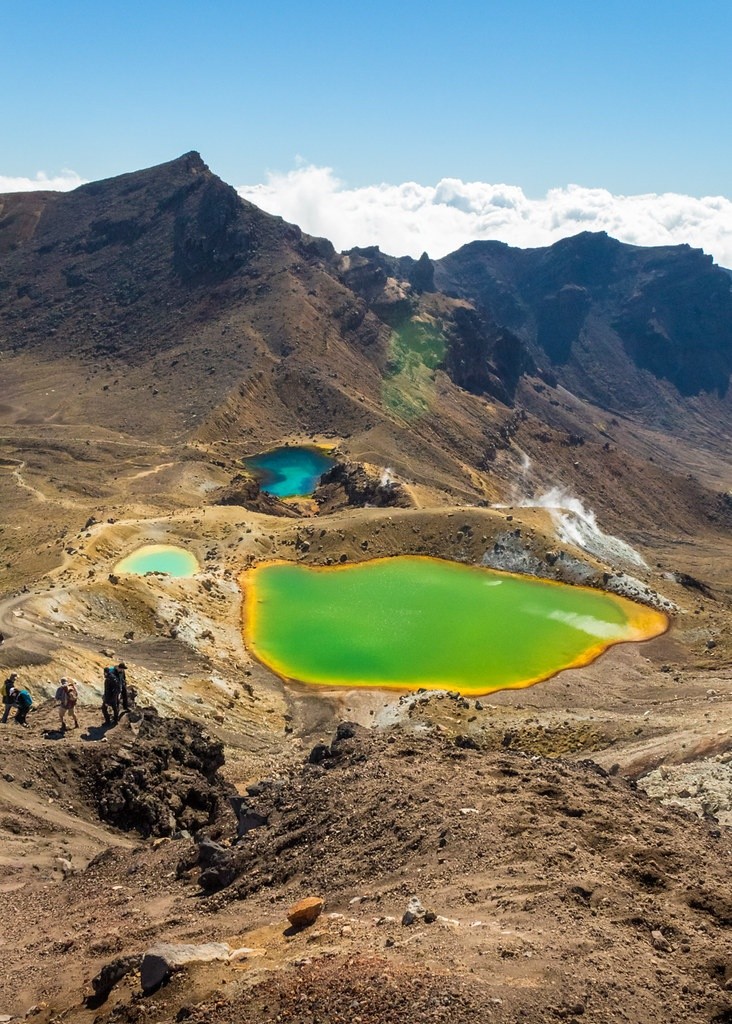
[123,707,130,711]
[111,716,118,720]
[60,726,66,730]
[75,722,78,728]
[102,721,111,726]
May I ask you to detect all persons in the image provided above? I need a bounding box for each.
[9,687,33,728]
[0,672,17,723]
[54,676,80,732]
[101,666,118,727]
[112,663,131,713]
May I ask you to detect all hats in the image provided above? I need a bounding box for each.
[10,672,18,678]
[9,687,16,696]
[118,663,128,669]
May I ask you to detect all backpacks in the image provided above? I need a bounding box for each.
[106,676,122,695]
[61,684,78,708]
[17,690,33,706]
